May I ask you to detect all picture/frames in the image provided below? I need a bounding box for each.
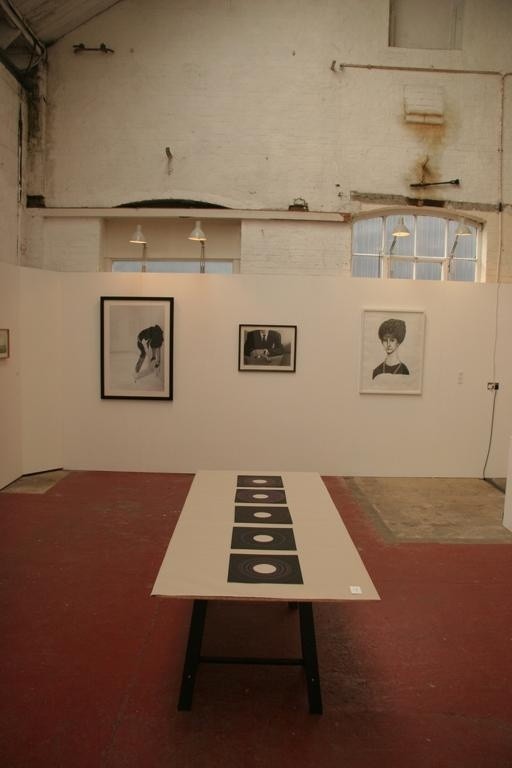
[237,324,297,372]
[100,296,174,400]
[1,328,9,359]
[358,307,427,396]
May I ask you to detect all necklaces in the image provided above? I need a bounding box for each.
[383,358,401,374]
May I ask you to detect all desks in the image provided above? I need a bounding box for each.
[149,470,382,716]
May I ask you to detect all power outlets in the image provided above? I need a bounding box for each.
[487,382,499,390]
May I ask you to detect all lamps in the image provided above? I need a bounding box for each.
[451,222,473,256]
[391,216,410,254]
[128,224,148,272]
[187,220,207,273]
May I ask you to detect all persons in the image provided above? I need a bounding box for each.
[244,330,283,356]
[132,325,163,392]
[372,317,409,379]
